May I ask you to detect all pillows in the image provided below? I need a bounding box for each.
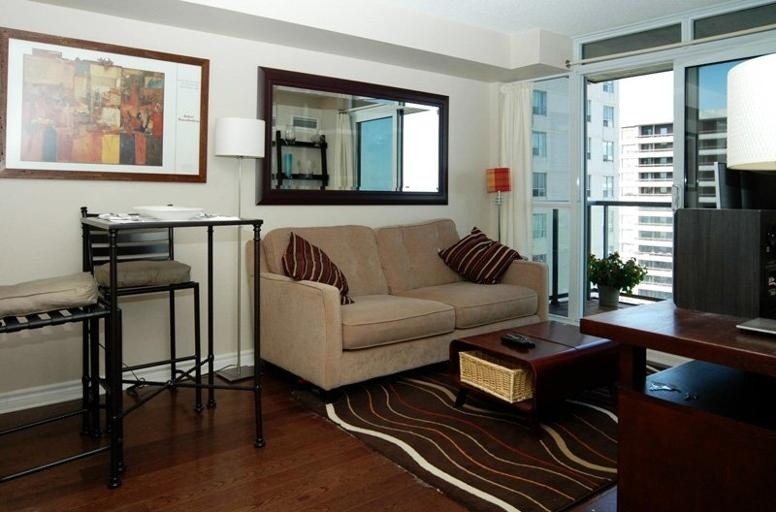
[436,226,517,284]
[282,233,354,307]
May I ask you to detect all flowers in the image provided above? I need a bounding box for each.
[586,251,648,294]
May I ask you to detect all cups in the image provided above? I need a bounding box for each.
[299,159,314,174]
[285,124,296,144]
[284,153,292,176]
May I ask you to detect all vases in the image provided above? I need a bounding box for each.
[597,284,620,307]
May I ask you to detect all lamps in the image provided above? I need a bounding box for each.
[215,117,265,384]
[484,166,512,245]
[726,52,776,334]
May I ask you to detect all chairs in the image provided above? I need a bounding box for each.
[0,271,126,484]
[78,202,205,432]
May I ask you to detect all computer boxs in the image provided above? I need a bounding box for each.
[673,207,768,320]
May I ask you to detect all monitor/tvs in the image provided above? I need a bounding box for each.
[713,161,775,280]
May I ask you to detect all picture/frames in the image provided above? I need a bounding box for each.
[0,27,211,183]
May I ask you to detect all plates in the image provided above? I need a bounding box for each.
[134,207,203,220]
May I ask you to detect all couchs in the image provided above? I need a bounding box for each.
[245,219,551,394]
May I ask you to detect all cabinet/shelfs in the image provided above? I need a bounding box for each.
[271,131,330,190]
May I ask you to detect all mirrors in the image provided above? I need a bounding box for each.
[255,65,449,207]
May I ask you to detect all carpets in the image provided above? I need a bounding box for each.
[292,360,678,511]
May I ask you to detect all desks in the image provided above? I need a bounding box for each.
[580,297,776,512]
[78,215,279,488]
